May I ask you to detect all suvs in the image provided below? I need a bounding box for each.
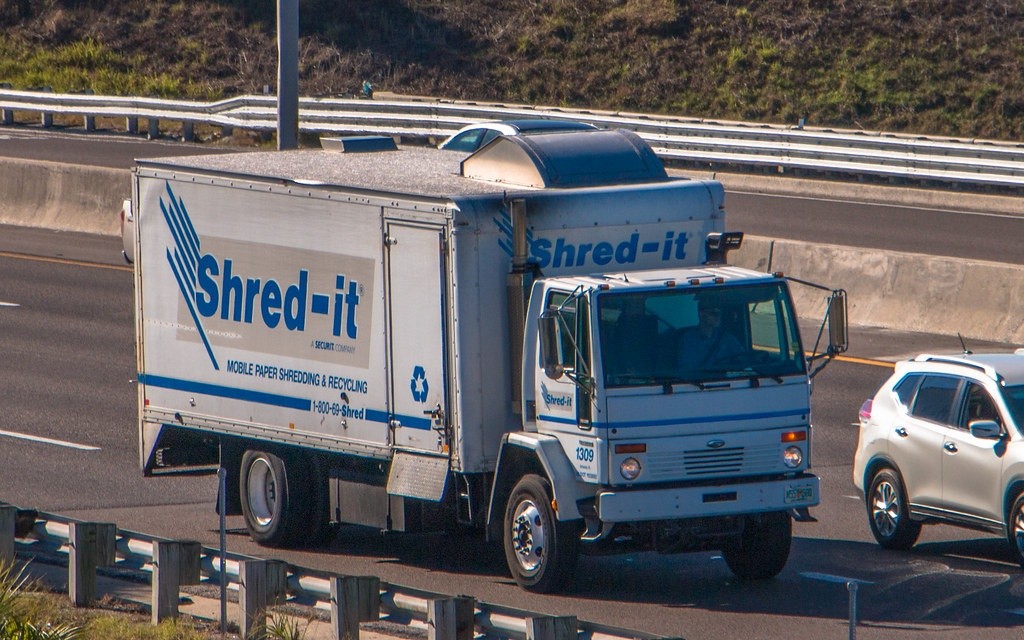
[851,348,1020,561]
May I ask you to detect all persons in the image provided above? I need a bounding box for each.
[677,300,752,368]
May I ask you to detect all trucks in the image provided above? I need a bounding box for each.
[120,118,850,595]
